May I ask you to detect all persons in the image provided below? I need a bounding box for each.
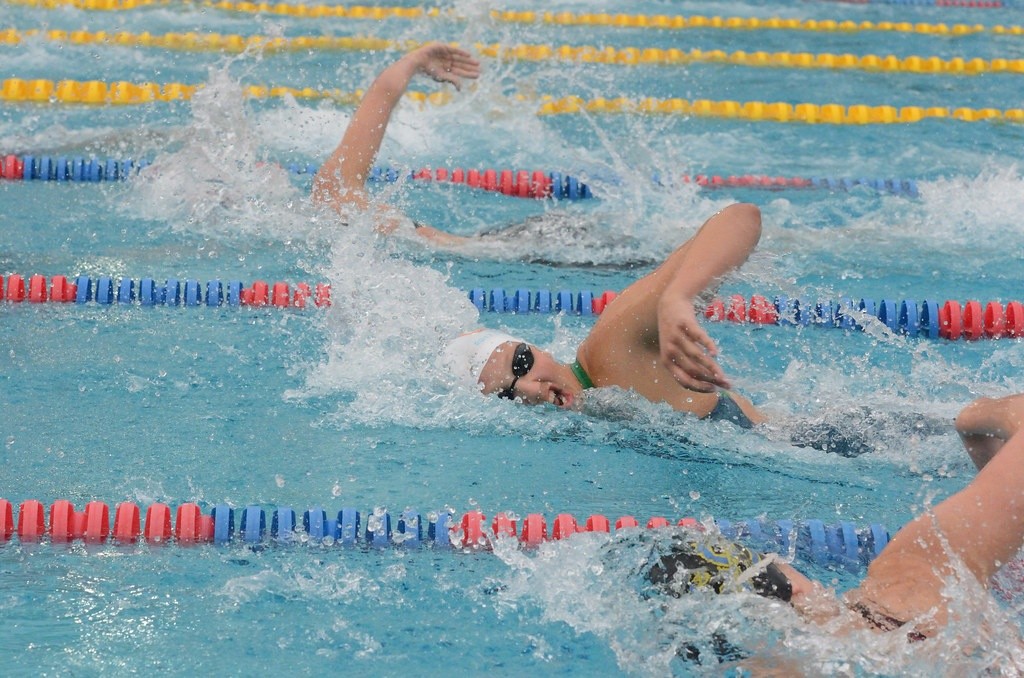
[648,395,1024,639]
[453,203,767,428]
[315,42,480,236]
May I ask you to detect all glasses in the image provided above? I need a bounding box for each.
[716,559,792,662]
[498,343,534,402]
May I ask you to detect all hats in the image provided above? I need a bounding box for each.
[628,530,750,646]
[442,329,513,392]
